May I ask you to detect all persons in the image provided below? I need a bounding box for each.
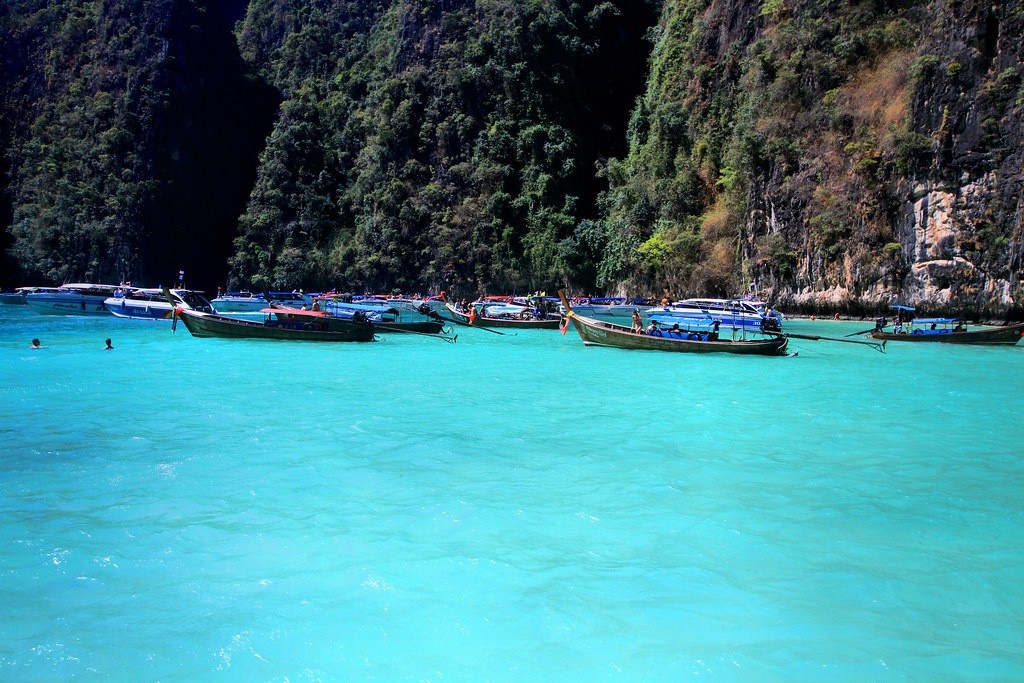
[652,293,657,308]
[103,339,114,350]
[311,299,320,311]
[931,323,936,330]
[301,303,307,311]
[628,297,633,305]
[589,292,592,304]
[575,296,579,304]
[30,339,40,348]
[709,318,720,337]
[662,296,668,306]
[955,322,963,331]
[645,320,661,334]
[672,324,680,332]
[480,304,486,317]
[455,299,477,324]
[630,309,643,334]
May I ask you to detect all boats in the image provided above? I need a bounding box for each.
[161,286,376,343]
[323,301,398,323]
[306,293,455,311]
[440,290,568,329]
[472,297,567,317]
[870,301,1024,346]
[105,289,220,321]
[644,298,785,331]
[221,291,260,299]
[25,282,131,316]
[260,289,444,335]
[568,298,656,317]
[0,286,59,305]
[212,291,315,312]
[558,289,788,355]
